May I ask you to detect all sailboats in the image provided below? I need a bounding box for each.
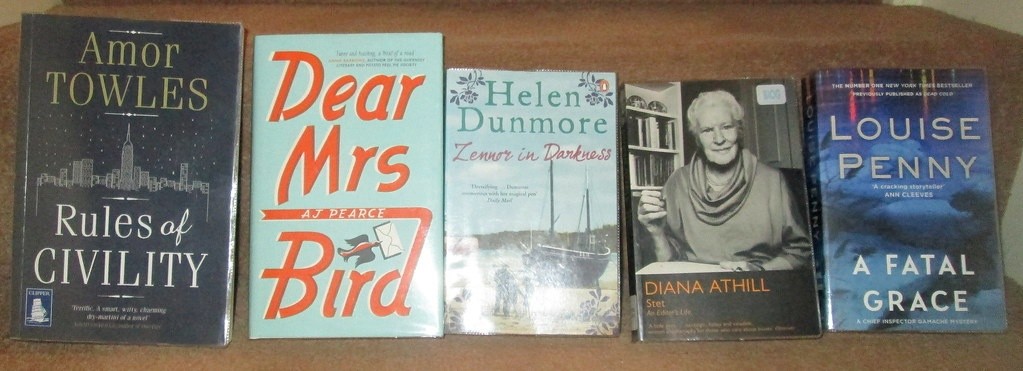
[517,157,611,288]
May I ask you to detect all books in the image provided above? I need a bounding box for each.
[248,30,444,340]
[12,11,242,348]
[629,111,678,189]
[617,76,823,340]
[799,64,1009,336]
[443,66,623,338]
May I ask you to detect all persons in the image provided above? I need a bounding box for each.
[636,91,809,274]
